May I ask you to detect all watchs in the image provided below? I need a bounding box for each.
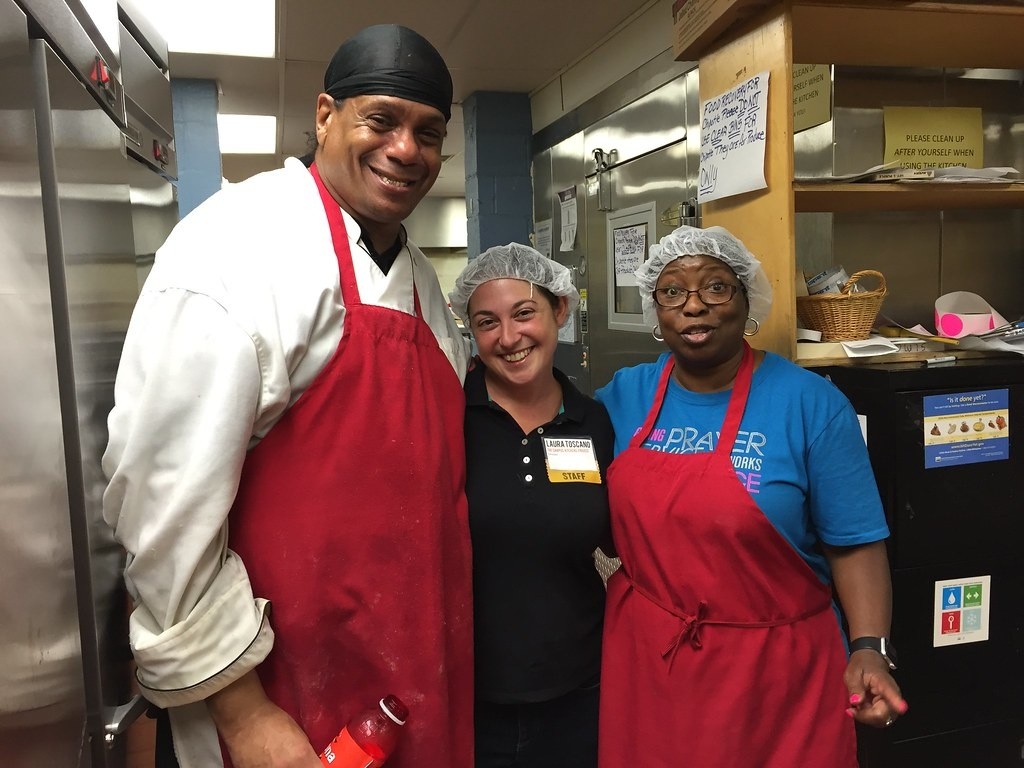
[851,635,901,670]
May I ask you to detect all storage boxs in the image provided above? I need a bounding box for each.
[669,0,749,61]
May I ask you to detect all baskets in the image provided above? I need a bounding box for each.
[797,271,889,344]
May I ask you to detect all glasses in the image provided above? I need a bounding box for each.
[652,283,745,308]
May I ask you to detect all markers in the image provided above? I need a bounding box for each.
[920,355,958,365]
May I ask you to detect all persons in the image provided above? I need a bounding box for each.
[592,226,911,768]
[448,240,615,768]
[100,21,480,768]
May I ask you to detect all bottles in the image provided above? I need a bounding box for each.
[318,694,408,768]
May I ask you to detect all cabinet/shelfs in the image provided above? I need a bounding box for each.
[698,0,1024,768]
